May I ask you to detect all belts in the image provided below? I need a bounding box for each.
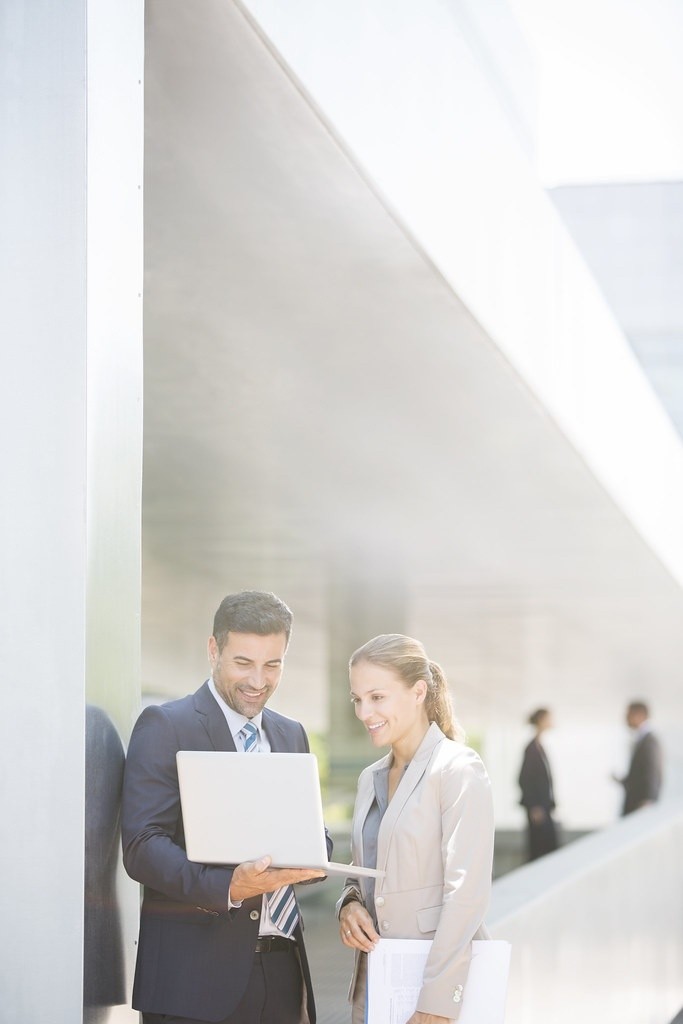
[247,937,293,955]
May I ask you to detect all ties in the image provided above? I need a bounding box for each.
[238,721,300,937]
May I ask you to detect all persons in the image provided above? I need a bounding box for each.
[519,708,559,861]
[336,633,495,1023]
[120,591,334,1024]
[620,703,662,816]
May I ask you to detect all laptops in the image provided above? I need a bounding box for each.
[176,751,385,878]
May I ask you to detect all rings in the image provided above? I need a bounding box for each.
[346,931,352,938]
